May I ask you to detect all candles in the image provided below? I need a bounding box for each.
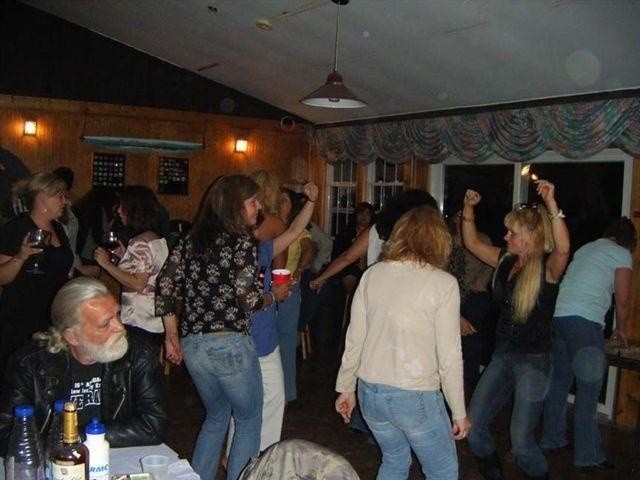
[0,440,202,480]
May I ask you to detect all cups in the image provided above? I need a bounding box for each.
[272,268,290,288]
[27,226,43,273]
[104,231,121,260]
[141,455,168,479]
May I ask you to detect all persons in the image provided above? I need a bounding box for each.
[444,206,494,335]
[249,170,289,271]
[540,216,635,471]
[0,276,171,458]
[309,189,439,293]
[54,166,79,253]
[93,184,170,348]
[221,181,319,477]
[0,169,74,359]
[276,187,312,410]
[336,204,472,480]
[155,173,318,480]
[298,193,332,333]
[462,180,570,480]
[331,201,377,291]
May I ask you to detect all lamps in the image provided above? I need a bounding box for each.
[299,0,369,109]
[24,113,37,136]
[235,131,248,153]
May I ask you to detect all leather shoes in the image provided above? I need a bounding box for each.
[593,463,615,473]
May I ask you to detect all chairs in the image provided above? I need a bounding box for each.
[237,438,359,480]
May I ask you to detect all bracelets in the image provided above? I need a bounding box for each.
[265,291,275,308]
[548,211,564,219]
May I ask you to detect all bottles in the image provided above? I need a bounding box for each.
[83,416,110,480]
[52,402,89,480]
[44,400,64,479]
[5,406,43,480]
